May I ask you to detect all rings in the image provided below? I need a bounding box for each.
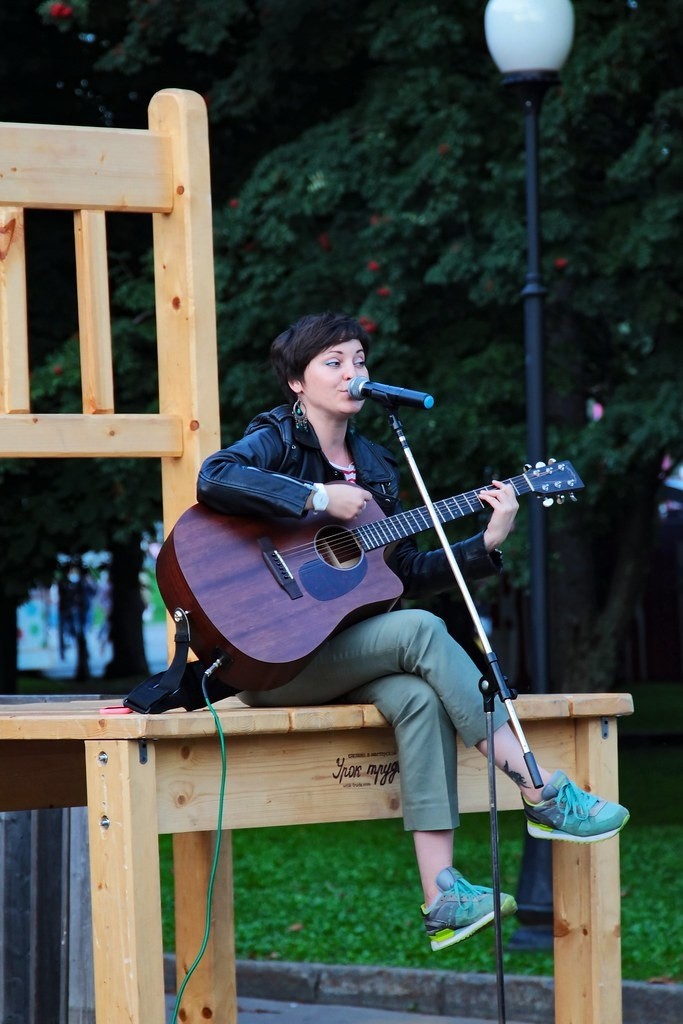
[362,501,367,510]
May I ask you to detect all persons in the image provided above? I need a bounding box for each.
[196,312,629,952]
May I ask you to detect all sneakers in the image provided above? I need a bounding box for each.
[420,865,519,952]
[522,769,631,844]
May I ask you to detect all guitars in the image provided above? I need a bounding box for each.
[154,456,587,692]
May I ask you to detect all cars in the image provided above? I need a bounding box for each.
[17,570,168,678]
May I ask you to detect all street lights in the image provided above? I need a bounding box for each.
[484,0,577,952]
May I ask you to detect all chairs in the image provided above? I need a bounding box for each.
[0,87,633,1024]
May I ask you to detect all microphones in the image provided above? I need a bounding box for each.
[348,376,435,410]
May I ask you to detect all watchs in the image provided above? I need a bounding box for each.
[311,482,328,517]
